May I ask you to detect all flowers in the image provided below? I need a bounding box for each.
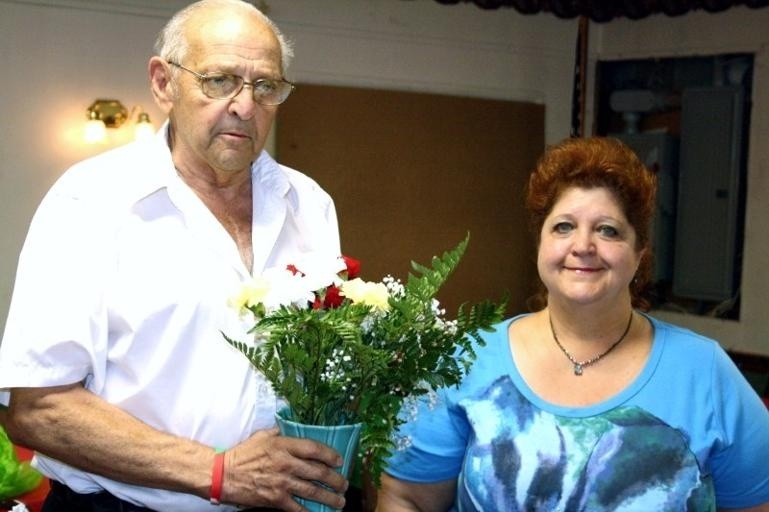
[222,232,513,490]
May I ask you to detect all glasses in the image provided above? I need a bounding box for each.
[166,60,295,108]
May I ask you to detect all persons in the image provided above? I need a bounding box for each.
[370,137,768,512]
[0,0,350,512]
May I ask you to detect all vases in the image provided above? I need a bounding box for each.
[271,404,364,512]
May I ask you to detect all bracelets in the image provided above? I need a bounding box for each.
[209,448,225,504]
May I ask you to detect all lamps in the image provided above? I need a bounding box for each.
[80,99,155,145]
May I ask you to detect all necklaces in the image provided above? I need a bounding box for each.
[546,314,633,376]
